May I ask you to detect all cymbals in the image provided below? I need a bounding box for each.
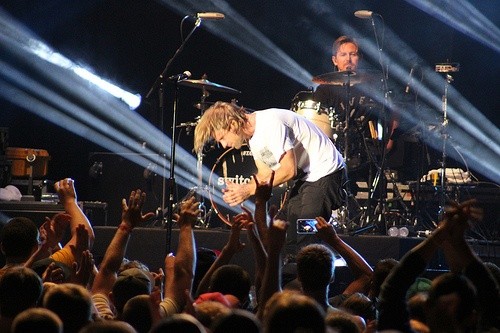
[312,68,381,86]
[178,79,242,94]
[435,63,460,73]
[195,101,255,112]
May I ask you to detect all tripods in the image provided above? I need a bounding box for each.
[331,22,487,241]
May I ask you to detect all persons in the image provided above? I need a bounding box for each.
[194,101,347,252]
[296,244,366,333]
[91,190,204,322]
[324,198,500,333]
[265,289,327,333]
[151,171,289,333]
[282,217,375,306]
[0,178,133,333]
[313,36,399,201]
[123,294,161,333]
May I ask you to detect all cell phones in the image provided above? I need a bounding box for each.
[297,219,318,234]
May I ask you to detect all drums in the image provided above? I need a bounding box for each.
[209,144,292,231]
[290,97,344,145]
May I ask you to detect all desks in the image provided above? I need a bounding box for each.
[81,201,108,226]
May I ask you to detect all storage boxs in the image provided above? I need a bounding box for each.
[4,147,51,177]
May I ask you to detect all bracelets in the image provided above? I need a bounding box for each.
[435,228,442,240]
[118,224,131,231]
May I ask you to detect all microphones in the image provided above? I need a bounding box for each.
[189,13,225,19]
[165,70,191,82]
[355,10,380,18]
